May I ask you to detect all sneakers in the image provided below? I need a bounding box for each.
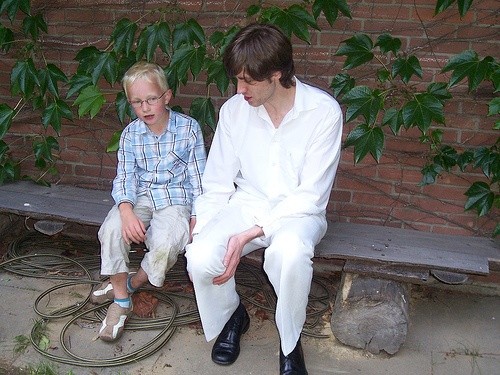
[90,274,133,304]
[99,302,133,341]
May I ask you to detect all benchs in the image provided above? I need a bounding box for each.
[0,179,500,355]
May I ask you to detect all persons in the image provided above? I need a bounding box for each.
[185,23,343,375]
[89,60,207,343]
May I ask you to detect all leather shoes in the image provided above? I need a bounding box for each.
[280,334,308,375]
[211,302,250,365]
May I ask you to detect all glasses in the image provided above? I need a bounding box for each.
[129,88,171,107]
